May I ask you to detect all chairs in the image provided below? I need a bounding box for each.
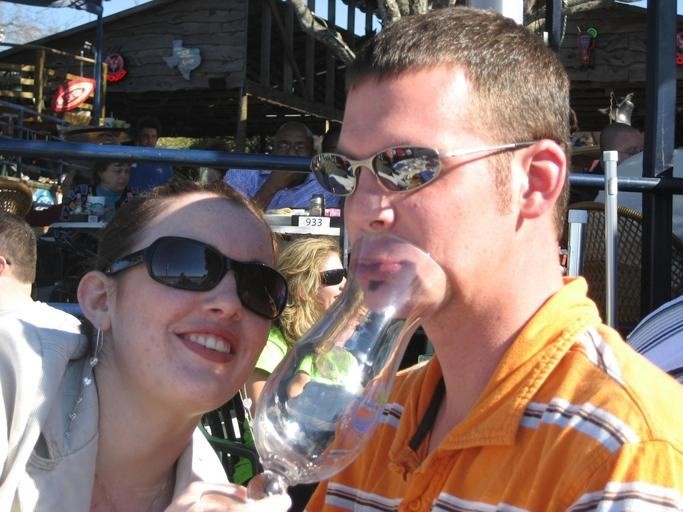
[559,201,683,338]
[0,178,33,219]
[197,386,263,489]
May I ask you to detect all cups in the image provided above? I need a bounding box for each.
[199,232,456,507]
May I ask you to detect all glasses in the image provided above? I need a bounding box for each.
[104,234,287,320]
[309,138,541,199]
[318,267,350,287]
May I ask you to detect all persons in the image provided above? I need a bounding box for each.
[163,6,683,512]
[223,119,341,211]
[53,119,161,303]
[566,102,682,379]
[233,238,366,512]
[192,130,228,186]
[0,209,39,296]
[0,180,278,512]
